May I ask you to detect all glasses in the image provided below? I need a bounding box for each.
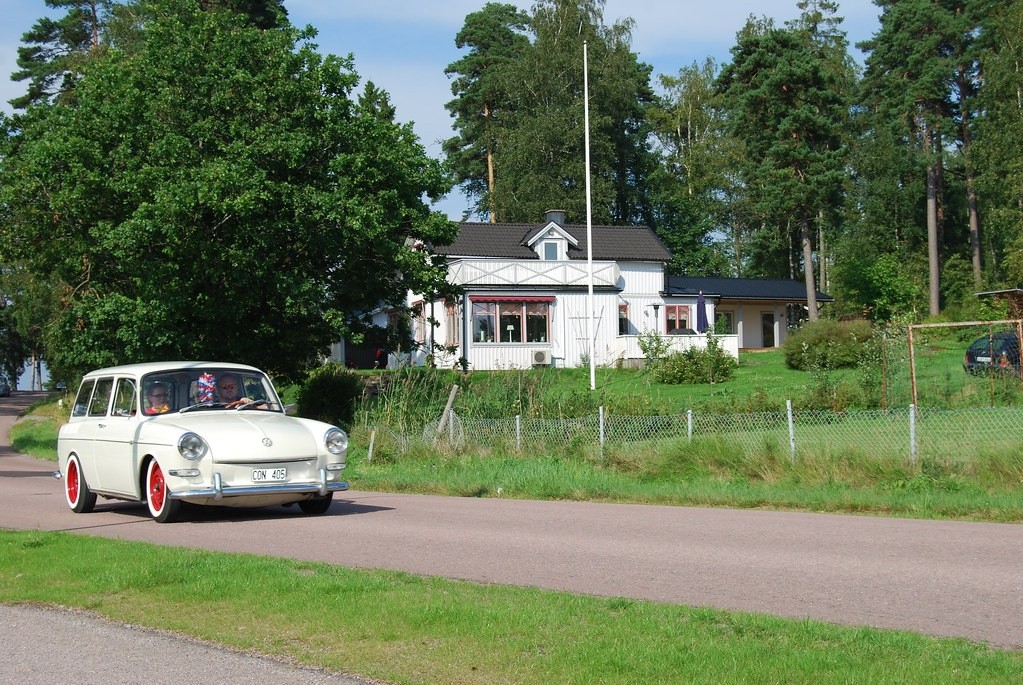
[219,384,238,390]
[150,393,168,398]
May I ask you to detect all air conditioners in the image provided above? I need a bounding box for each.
[531,348,552,365]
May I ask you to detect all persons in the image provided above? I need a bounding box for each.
[215,374,269,410]
[144,382,173,415]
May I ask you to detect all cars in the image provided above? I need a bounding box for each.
[54,361,351,524]
[963,328,1021,380]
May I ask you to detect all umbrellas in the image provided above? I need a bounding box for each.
[697,291,709,334]
